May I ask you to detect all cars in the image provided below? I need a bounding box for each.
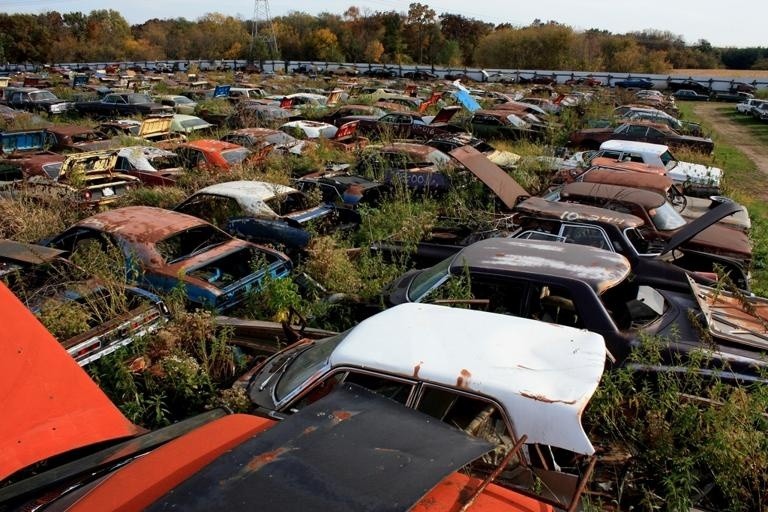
[736,98,767,116]
[751,102,768,121]
[170,180,339,251]
[0,63,753,292]
[40,204,296,317]
[378,235,768,390]
[670,88,711,102]
[0,278,558,512]
[246,297,640,512]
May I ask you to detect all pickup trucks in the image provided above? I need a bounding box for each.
[668,80,713,94]
